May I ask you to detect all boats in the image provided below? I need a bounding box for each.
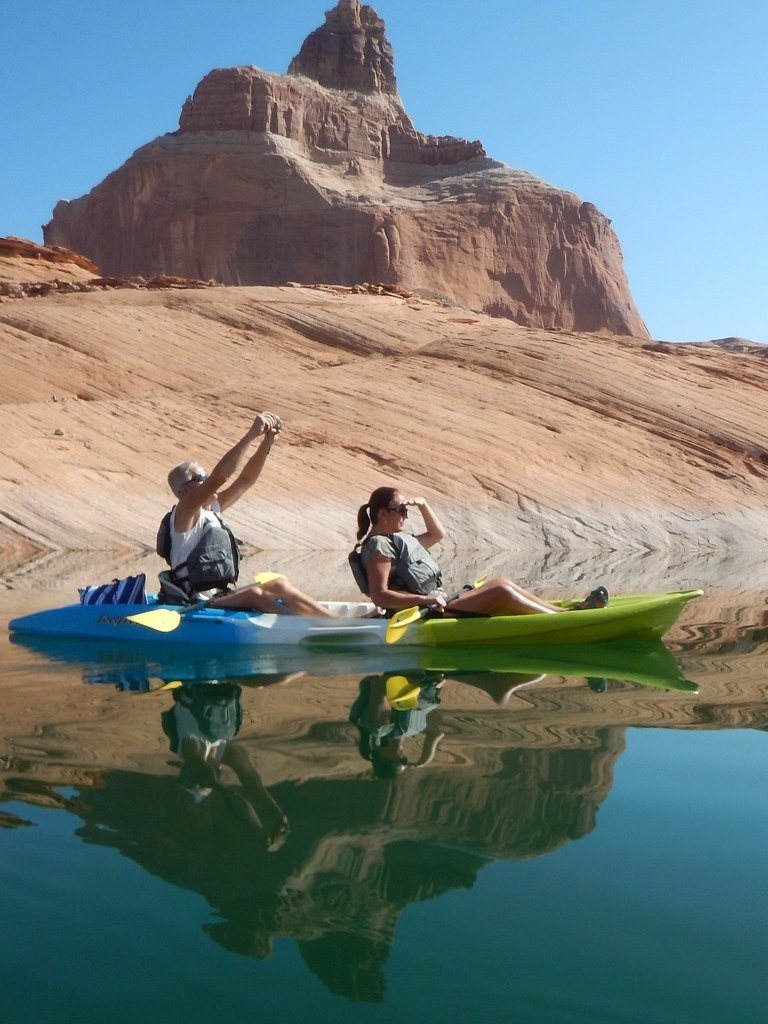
[8,587,702,644]
[7,633,699,694]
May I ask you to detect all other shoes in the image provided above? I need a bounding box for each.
[576,586,610,609]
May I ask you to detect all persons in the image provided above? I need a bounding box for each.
[348,670,608,782]
[348,486,609,619]
[167,411,382,619]
[160,670,306,854]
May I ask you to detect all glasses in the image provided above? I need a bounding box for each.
[387,503,408,519]
[178,473,210,494]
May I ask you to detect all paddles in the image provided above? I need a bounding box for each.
[384,573,488,645]
[384,669,483,713]
[129,677,239,697]
[126,569,288,633]
